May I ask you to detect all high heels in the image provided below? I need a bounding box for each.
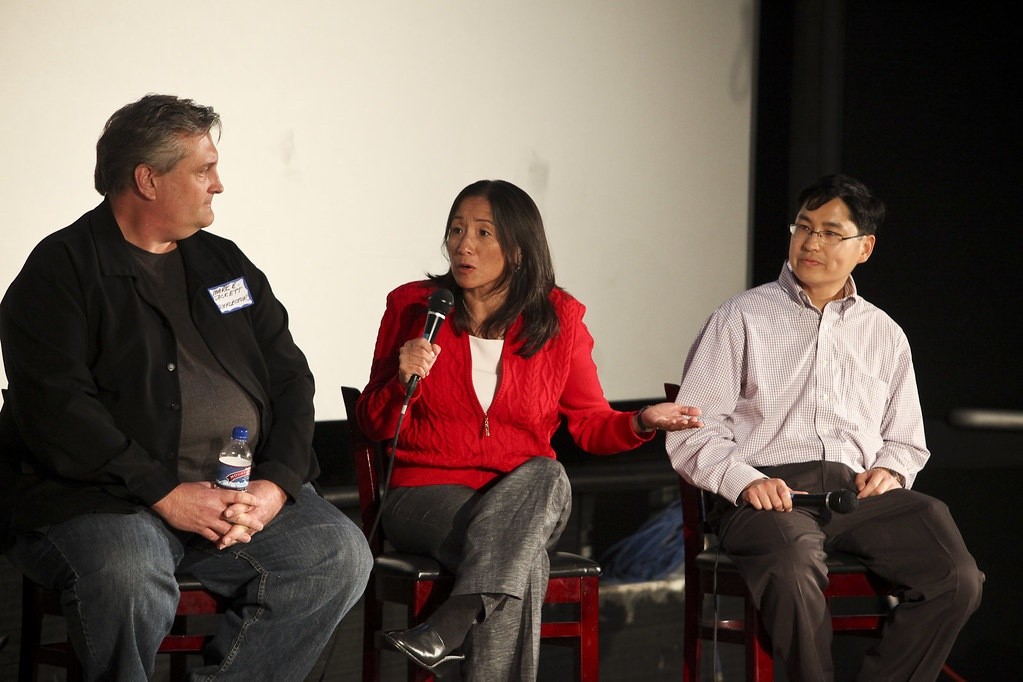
[383,623,467,679]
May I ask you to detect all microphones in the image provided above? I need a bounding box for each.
[788,489,858,514]
[407,289,454,398]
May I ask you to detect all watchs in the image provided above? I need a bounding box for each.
[888,469,905,488]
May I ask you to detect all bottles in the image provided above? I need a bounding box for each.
[214,424,253,495]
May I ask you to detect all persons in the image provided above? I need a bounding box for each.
[356,181,704,682]
[665,178,986,682]
[0,94,374,682]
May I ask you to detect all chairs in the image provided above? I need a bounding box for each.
[664,382,896,682]
[338,385,602,682]
[3,388,231,682]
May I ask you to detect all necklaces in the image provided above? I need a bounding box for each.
[463,300,480,326]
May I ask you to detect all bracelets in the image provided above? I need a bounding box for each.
[638,405,661,431]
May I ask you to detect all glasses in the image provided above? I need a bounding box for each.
[787,223,867,246]
